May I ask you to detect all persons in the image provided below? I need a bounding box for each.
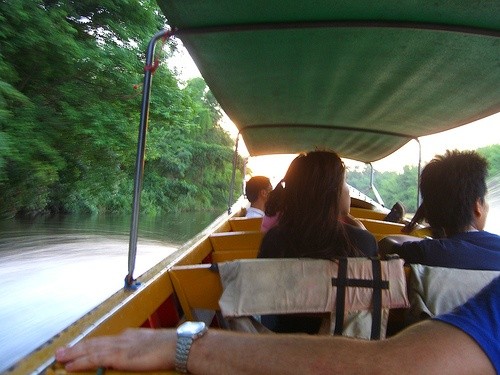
[245,176,287,233]
[55,267,500,375]
[390,149,500,270]
[255,147,378,260]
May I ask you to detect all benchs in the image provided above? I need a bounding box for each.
[167,197,432,321]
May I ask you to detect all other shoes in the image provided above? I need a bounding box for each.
[385,201,405,223]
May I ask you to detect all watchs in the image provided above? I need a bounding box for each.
[175,320,208,374]
